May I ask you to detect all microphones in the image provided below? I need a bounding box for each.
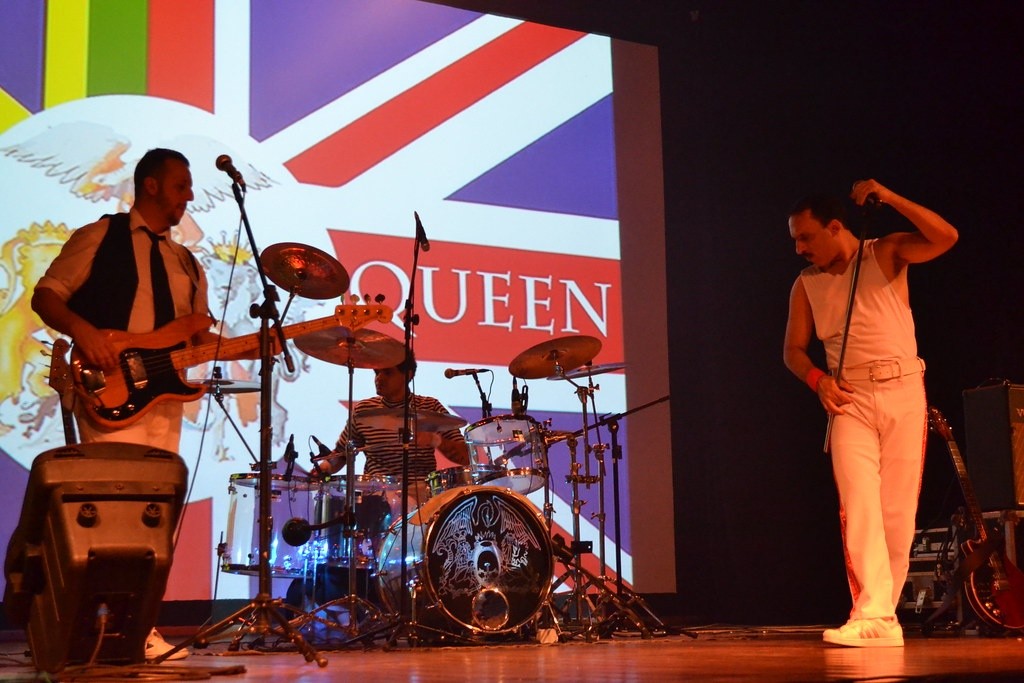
[217,155,246,187]
[311,435,333,457]
[284,434,295,462]
[414,211,431,251]
[512,377,522,414]
[494,443,527,465]
[445,368,489,379]
[853,180,879,206]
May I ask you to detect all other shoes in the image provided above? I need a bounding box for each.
[145,627,189,660]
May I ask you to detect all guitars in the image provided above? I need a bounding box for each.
[38,336,78,446]
[67,292,394,434]
[925,405,1024,633]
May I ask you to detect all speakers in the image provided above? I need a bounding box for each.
[962,384,1024,511]
[0,442,188,679]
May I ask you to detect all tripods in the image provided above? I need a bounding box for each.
[542,364,700,647]
[152,182,488,669]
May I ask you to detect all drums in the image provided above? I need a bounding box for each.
[378,485,555,635]
[426,464,508,498]
[219,472,323,580]
[314,473,402,570]
[463,414,547,495]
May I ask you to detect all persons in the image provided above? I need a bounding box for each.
[30,147,285,660]
[309,345,470,520]
[784,180,958,648]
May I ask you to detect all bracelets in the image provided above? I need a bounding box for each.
[806,367,825,393]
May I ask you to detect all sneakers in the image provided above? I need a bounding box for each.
[822,614,904,647]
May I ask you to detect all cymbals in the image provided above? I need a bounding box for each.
[546,361,631,381]
[293,327,407,370]
[544,429,584,441]
[508,334,603,380]
[352,406,468,433]
[260,241,350,300]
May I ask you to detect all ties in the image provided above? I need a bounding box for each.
[141,226,175,329]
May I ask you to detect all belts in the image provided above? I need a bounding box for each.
[832,360,922,382]
